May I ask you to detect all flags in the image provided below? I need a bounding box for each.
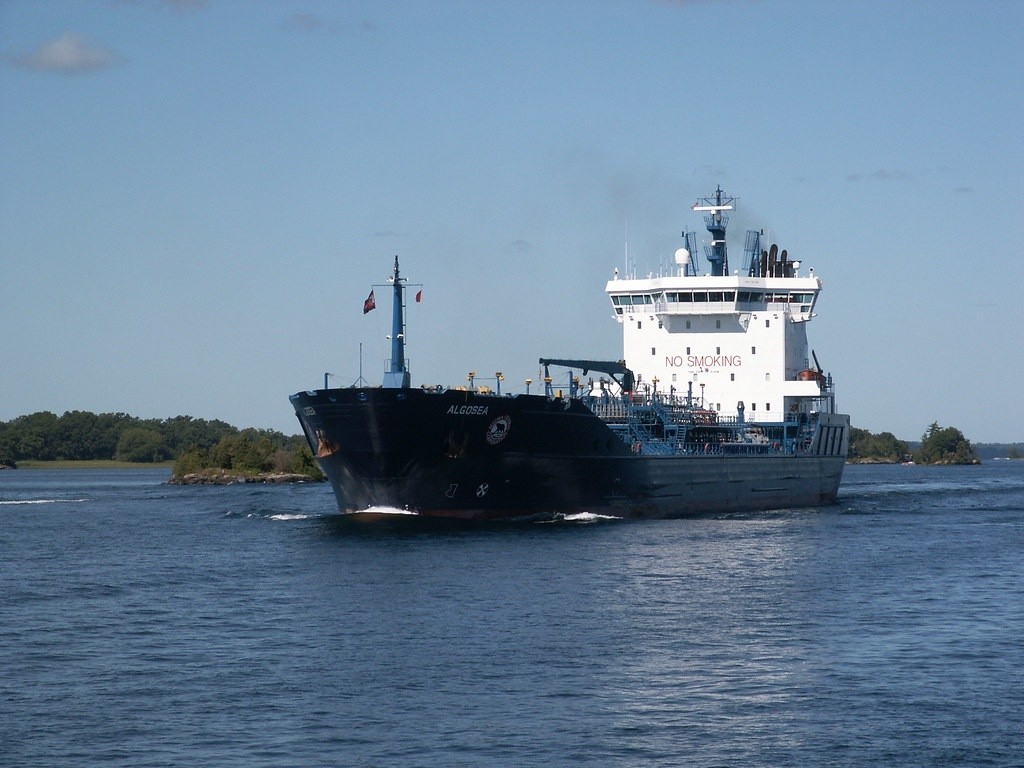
[416,289,421,303]
[363,289,376,315]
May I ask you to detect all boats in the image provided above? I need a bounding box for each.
[288,183,853,521]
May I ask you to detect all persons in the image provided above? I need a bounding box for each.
[611,391,815,456]
